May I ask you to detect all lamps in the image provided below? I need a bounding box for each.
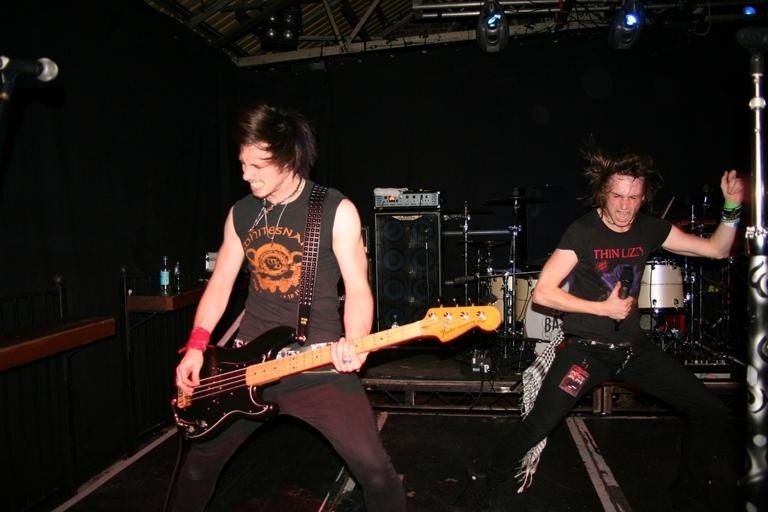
[610,2,643,52]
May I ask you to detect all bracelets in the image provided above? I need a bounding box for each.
[719,199,744,229]
[177,327,211,353]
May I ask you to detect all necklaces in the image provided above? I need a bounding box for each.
[260,198,289,247]
[248,177,302,231]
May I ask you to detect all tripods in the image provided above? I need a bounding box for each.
[668,247,737,362]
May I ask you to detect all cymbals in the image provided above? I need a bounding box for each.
[672,219,720,226]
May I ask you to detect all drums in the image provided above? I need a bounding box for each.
[523,280,570,357]
[488,274,538,323]
[639,310,686,340]
[637,256,685,313]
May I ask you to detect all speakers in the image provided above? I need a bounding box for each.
[375,210,442,351]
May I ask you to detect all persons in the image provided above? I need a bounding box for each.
[169,102,405,511]
[451,142,747,512]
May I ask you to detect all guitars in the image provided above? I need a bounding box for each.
[171,301,501,441]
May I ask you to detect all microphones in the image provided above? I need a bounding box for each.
[614,268,634,329]
[455,274,480,285]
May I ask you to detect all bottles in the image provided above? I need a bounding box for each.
[159,255,173,297]
[390,315,401,349]
[174,256,186,296]
